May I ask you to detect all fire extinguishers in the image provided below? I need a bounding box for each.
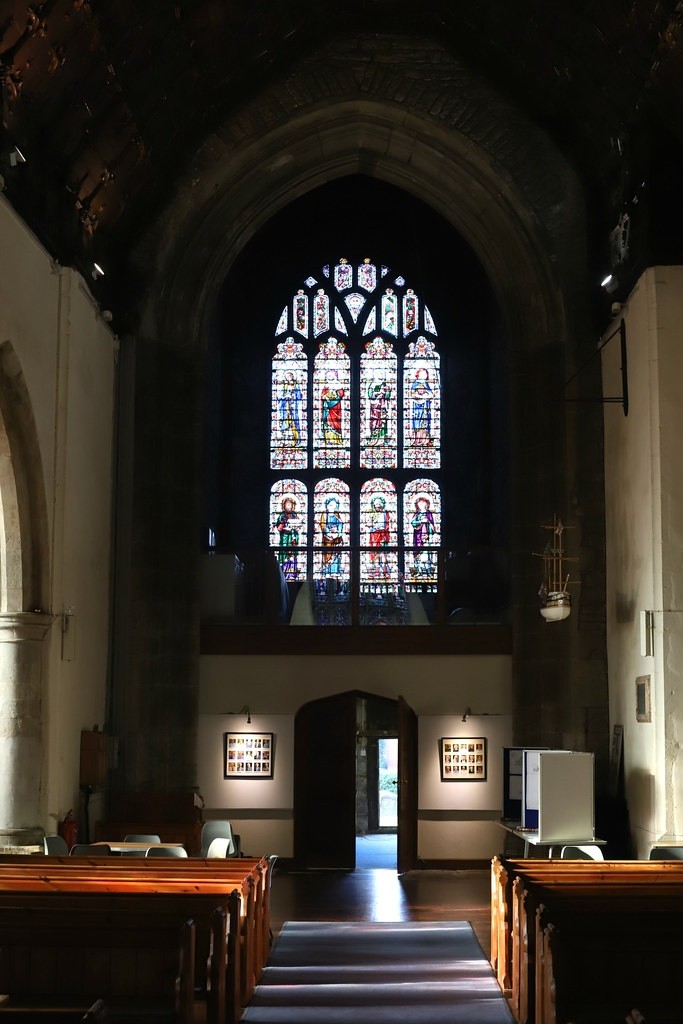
[60,809,78,851]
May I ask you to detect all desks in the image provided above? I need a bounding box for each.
[95,842,184,856]
[495,820,606,859]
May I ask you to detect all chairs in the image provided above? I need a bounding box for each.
[561,846,605,860]
[69,844,111,857]
[650,848,683,860]
[145,847,188,859]
[44,835,68,855]
[199,820,236,859]
[120,835,161,857]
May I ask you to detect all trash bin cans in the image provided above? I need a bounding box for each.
[234,835,240,857]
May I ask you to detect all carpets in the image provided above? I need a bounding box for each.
[239,920,516,1024]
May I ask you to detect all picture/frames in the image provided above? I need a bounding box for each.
[224,731,273,777]
[636,675,650,723]
[439,737,487,782]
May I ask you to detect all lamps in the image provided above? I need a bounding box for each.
[242,706,252,723]
[611,300,629,313]
[92,262,105,280]
[538,513,581,623]
[462,706,472,722]
[601,273,621,292]
[6,145,26,166]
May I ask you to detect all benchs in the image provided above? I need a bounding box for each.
[490,857,683,1024]
[0,853,275,1024]
